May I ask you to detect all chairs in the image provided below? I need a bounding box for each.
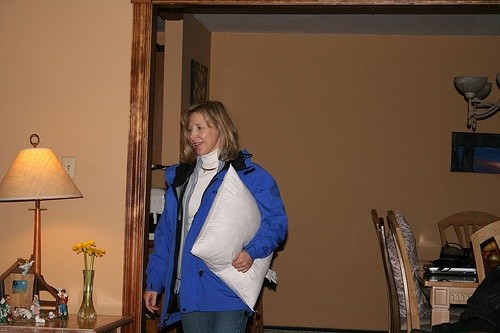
[438,211,500,248]
[471,220,500,285]
[372,208,464,333]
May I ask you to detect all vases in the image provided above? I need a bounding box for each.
[77,270,97,329]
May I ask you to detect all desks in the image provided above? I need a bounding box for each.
[0,134,84,322]
[0,314,133,333]
[415,271,478,331]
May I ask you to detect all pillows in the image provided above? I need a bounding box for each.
[190,164,278,312]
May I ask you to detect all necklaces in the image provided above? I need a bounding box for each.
[201,164,216,172]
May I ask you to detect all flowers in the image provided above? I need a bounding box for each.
[72,240,106,307]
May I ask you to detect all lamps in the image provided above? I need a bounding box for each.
[454,73,500,130]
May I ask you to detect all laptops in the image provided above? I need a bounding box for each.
[424,263,476,276]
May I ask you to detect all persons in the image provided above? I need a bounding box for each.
[31,295,40,316]
[1,294,13,321]
[145,101,287,333]
[59,289,68,316]
[459,264,500,333]
